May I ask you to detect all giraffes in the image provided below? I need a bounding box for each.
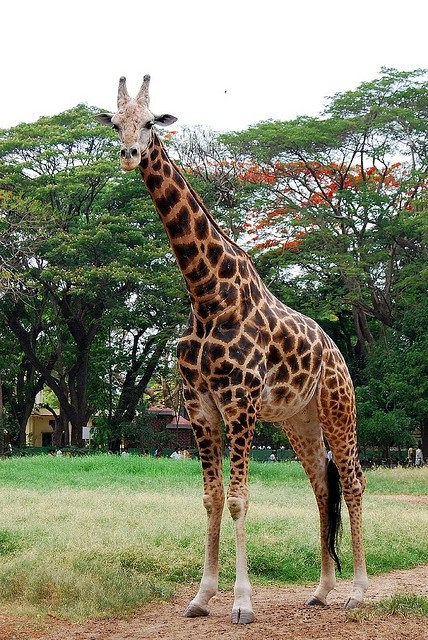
[89,74,369,624]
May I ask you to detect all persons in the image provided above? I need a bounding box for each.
[56,447,63,455]
[144,450,149,456]
[122,447,129,456]
[415,445,425,468]
[170,447,190,459]
[269,451,276,461]
[408,446,415,467]
[48,448,56,456]
[154,448,163,458]
